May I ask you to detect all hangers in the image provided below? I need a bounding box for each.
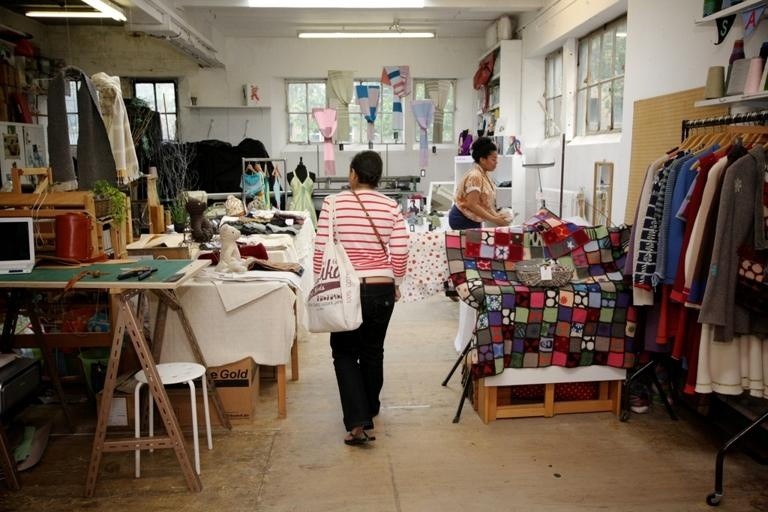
[656,110,767,174]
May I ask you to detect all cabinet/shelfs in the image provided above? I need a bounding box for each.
[453,155,526,232]
[693,0,767,108]
[471,39,521,139]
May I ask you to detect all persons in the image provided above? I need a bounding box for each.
[286,154,316,231]
[445,135,514,301]
[311,150,408,448]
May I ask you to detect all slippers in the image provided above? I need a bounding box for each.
[344,419,376,442]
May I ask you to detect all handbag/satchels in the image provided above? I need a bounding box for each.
[306,242,363,333]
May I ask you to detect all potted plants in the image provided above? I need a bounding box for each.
[168,200,189,232]
[87,178,127,217]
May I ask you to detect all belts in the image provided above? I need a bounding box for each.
[358,277,395,283]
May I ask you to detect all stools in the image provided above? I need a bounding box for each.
[128,362,233,478]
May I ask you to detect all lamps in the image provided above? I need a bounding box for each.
[294,21,438,40]
[23,0,127,24]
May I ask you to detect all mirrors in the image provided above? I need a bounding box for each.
[427,180,455,216]
[592,163,614,227]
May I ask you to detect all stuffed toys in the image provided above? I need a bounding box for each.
[214,223,248,274]
[184,197,214,243]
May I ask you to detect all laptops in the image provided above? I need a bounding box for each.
[0,217,37,275]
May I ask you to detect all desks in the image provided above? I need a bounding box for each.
[193,210,311,344]
[126,264,300,420]
[1,257,214,501]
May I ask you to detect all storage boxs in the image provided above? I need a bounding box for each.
[95,369,142,430]
[164,356,259,419]
[240,82,262,106]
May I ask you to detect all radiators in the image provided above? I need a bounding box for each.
[538,187,584,220]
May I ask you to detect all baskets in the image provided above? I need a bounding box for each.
[515,258,573,287]
